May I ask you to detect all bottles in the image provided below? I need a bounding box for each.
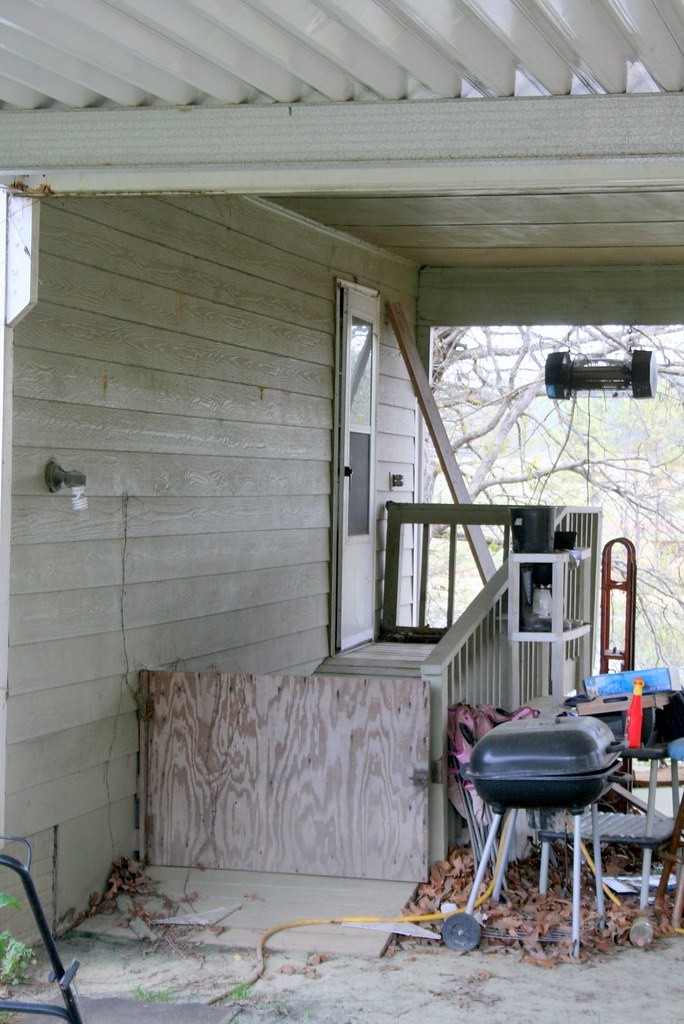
[630,916,653,947]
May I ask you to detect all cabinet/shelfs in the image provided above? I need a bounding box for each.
[510,546,593,716]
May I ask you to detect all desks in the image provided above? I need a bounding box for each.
[537,740,680,913]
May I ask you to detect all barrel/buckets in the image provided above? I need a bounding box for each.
[509,507,556,554]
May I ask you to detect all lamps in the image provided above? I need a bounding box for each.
[45,462,88,512]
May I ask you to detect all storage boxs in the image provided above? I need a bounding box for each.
[583,664,682,701]
[574,693,671,749]
